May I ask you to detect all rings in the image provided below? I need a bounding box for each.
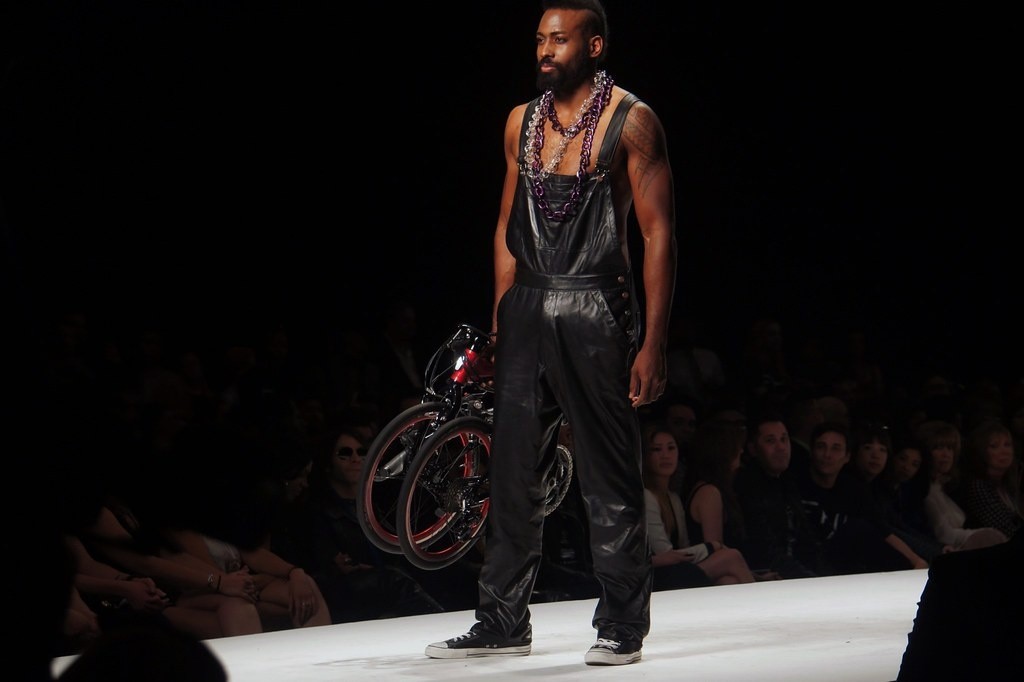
[345,558,348,561]
[657,393,663,400]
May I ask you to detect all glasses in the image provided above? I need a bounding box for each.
[333,447,368,457]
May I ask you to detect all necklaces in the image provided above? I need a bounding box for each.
[524,70,613,222]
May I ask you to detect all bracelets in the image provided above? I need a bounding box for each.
[207,573,213,591]
[128,573,138,581]
[489,333,497,336]
[216,576,222,592]
[288,567,300,573]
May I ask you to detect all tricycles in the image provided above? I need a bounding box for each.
[356,325,573,571]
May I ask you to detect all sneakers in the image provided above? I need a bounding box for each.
[584,637,642,664]
[424,621,531,659]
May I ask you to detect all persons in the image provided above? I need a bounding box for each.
[643,315,1024,589]
[0,303,422,682]
[424,0,675,667]
